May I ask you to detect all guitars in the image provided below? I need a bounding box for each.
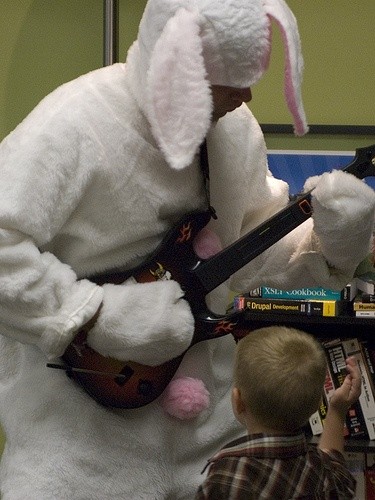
[60,143,375,410]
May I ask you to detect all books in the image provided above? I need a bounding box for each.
[232,272,375,500]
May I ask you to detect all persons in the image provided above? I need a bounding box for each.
[194,325,363,500]
[1,0,374,500]
